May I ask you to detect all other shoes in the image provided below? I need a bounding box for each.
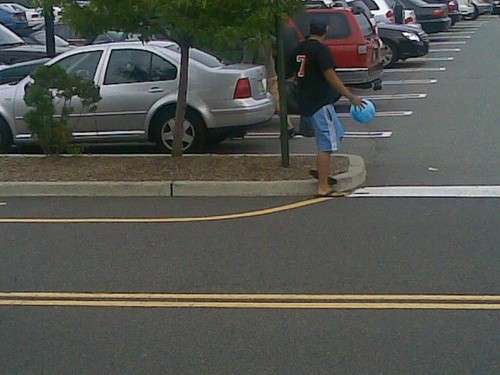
[280,127,297,139]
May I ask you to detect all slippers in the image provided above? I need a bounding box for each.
[314,190,345,197]
[309,169,338,185]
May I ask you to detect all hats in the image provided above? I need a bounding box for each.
[310,19,326,35]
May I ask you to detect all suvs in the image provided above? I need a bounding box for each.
[280,5,386,88]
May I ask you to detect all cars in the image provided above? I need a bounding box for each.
[0,2,277,155]
[304,0,500,70]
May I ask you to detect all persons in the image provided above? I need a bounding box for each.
[242,33,294,131]
[291,19,368,197]
